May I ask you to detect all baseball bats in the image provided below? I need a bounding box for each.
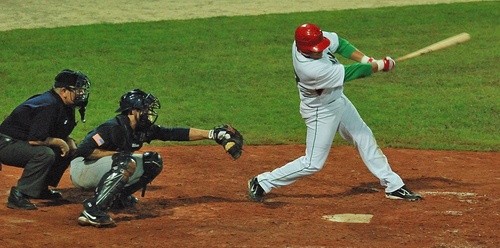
[394,32,473,63]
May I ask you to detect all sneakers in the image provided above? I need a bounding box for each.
[78,209,115,225]
[385,185,422,201]
[247,177,263,201]
[111,194,138,207]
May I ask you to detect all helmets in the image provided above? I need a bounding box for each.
[114,88,161,131]
[295,23,330,54]
[54,68,90,123]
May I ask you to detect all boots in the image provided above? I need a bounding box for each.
[35,187,62,198]
[7,186,38,209]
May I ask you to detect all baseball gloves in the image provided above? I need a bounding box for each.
[212,120,244,161]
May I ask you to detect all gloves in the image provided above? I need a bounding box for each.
[360,54,395,72]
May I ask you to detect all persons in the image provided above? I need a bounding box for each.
[248,24,418,209]
[69,90,236,227]
[1,68,91,211]
